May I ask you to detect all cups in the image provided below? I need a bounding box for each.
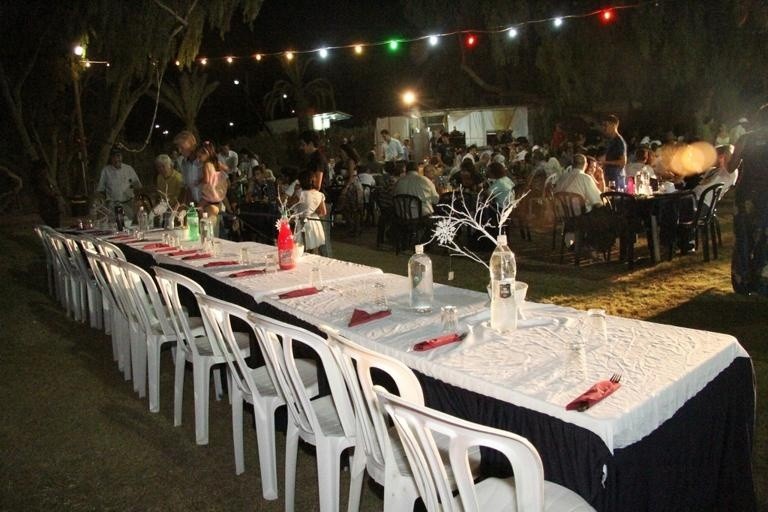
[585,309,609,347]
[238,248,459,334]
[77,220,95,230]
[121,224,179,248]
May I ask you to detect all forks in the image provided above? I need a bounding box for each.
[386,308,392,313]
[577,374,623,415]
[455,332,470,340]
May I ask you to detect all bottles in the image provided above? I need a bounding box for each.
[408,245,434,312]
[113,200,213,253]
[609,171,657,195]
[277,210,296,271]
[488,235,518,331]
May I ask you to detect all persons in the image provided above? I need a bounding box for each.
[97,130,275,242]
[286,168,330,257]
[340,129,515,250]
[722,102,768,300]
[300,130,334,258]
[516,114,749,248]
